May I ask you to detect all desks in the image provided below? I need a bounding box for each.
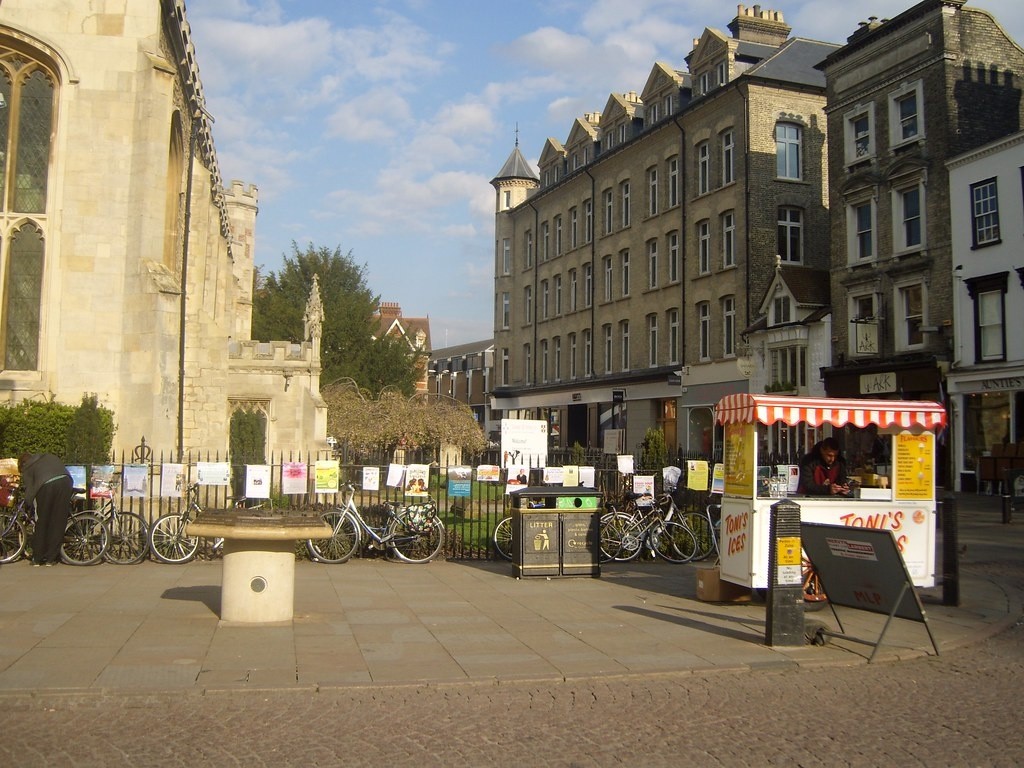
[185,507,333,621]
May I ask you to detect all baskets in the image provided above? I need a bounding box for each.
[671,488,691,508]
[0,486,19,508]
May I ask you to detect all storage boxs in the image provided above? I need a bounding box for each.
[696,567,752,602]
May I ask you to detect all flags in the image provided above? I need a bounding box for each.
[936,376,949,445]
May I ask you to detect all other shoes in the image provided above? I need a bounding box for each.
[46,560,53,566]
[29,560,40,567]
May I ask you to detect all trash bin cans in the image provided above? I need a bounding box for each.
[509,487,603,579]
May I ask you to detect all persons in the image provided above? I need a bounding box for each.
[517,469,526,484]
[405,479,416,491]
[18,453,73,568]
[418,479,427,491]
[796,436,851,496]
[504,451,508,468]
[797,444,806,463]
[860,427,886,462]
[669,441,684,468]
[768,445,790,465]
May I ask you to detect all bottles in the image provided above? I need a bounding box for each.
[769,473,789,498]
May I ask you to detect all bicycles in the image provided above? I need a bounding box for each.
[148,481,265,564]
[0,478,152,567]
[307,478,446,564]
[491,487,717,565]
